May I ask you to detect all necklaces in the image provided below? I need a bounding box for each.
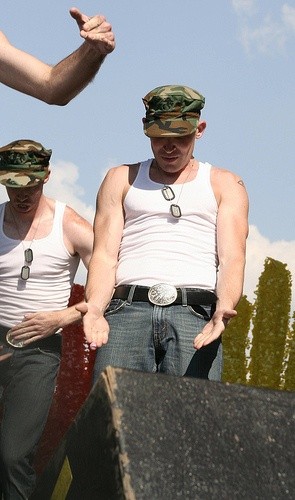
[157,163,194,218]
[8,204,44,280]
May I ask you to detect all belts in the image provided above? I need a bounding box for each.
[113,285,219,305]
[0,327,51,350]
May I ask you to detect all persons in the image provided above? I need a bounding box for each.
[1,7,115,107]
[0,139,94,500]
[74,86,248,380]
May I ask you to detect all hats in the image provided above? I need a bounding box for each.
[142,84,205,138]
[0,139,52,188]
